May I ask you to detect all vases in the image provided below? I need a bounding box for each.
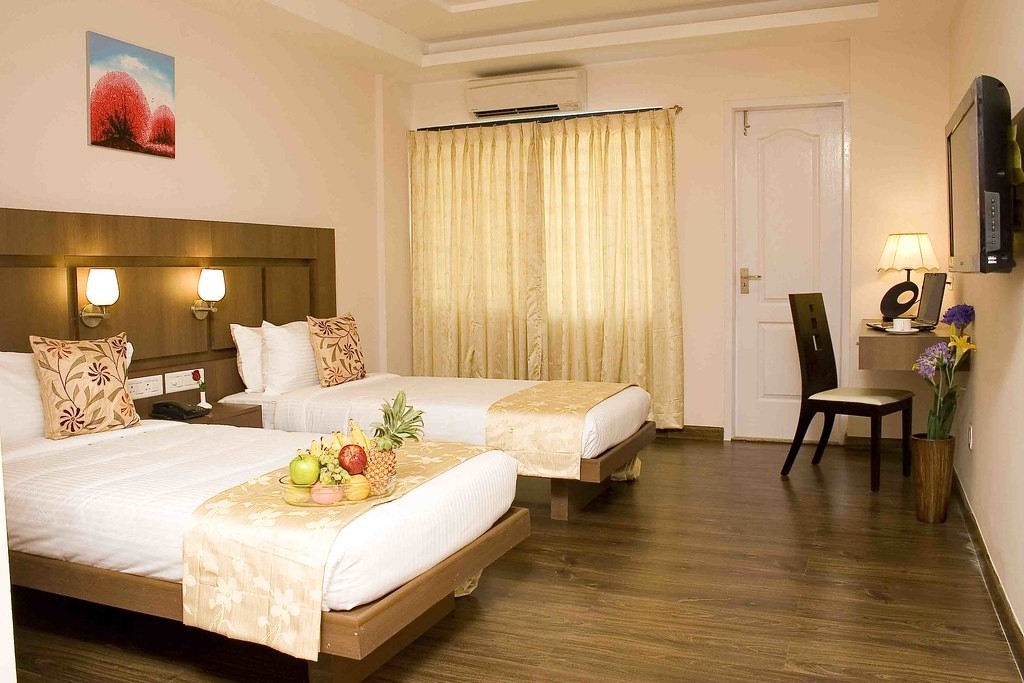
[197,390,212,409]
[913,432,955,525]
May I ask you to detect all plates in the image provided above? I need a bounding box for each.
[885,328,919,334]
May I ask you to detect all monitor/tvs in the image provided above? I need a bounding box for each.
[944,72,1016,276]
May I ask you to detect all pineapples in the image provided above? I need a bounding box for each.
[363,392,425,496]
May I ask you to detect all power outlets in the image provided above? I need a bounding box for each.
[124,375,164,401]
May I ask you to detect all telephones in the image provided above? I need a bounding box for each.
[152,400,210,420]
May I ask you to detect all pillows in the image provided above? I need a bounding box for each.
[0,332,141,444]
[227,314,365,394]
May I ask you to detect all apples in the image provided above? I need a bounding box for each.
[286,445,369,504]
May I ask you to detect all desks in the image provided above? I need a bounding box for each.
[859,318,970,371]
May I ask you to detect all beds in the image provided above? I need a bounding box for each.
[3,416,533,683]
[219,373,654,523]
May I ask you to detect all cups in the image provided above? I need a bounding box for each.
[893,319,911,330]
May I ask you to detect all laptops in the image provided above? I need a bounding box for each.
[866,272,948,331]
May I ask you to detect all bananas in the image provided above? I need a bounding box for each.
[297,418,370,457]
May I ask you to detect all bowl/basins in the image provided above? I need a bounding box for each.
[279,471,400,506]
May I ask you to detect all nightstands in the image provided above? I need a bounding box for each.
[147,401,262,429]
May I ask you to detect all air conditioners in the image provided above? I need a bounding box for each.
[467,68,585,118]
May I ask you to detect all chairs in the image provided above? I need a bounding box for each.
[779,293,913,492]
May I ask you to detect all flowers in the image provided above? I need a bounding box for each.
[192,370,206,392]
[912,305,976,439]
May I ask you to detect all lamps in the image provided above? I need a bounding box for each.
[191,267,225,320]
[876,233,938,317]
[80,267,121,329]
